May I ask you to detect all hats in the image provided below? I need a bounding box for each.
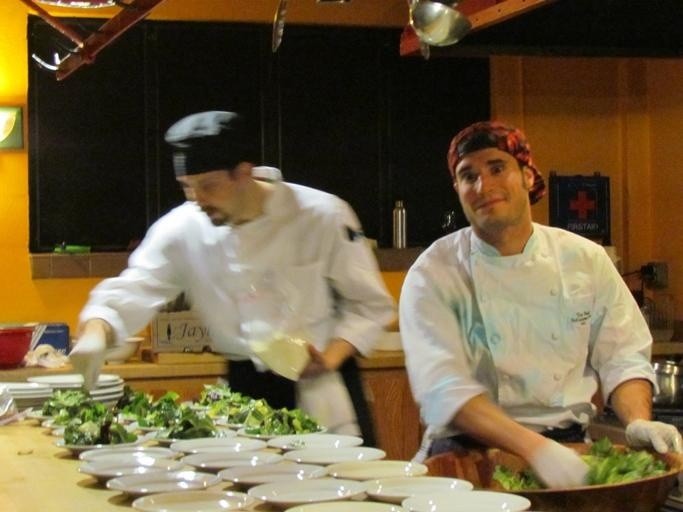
[165,110,258,176]
[447,121,545,205]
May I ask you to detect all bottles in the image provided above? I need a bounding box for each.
[392,200,407,250]
[641,294,674,343]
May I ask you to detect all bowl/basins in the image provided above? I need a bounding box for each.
[422,443,682,512]
[0,326,34,370]
[103,337,144,365]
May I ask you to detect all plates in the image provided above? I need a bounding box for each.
[215,463,328,487]
[216,414,246,429]
[264,433,364,449]
[283,447,387,464]
[77,446,174,472]
[143,427,235,443]
[77,463,196,483]
[361,476,474,503]
[235,425,329,441]
[327,459,431,481]
[26,376,125,414]
[130,489,253,510]
[103,471,219,496]
[0,378,54,418]
[168,436,268,454]
[41,416,122,431]
[180,450,284,473]
[248,478,366,505]
[56,431,150,450]
[400,492,531,512]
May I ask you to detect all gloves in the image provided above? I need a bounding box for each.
[624,418,683,455]
[534,439,591,488]
[70,332,107,392]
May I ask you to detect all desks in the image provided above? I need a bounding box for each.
[0,338,682,512]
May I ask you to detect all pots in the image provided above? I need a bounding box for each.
[649,359,681,408]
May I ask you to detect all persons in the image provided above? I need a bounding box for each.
[398,122,683,488]
[68,109,398,449]
